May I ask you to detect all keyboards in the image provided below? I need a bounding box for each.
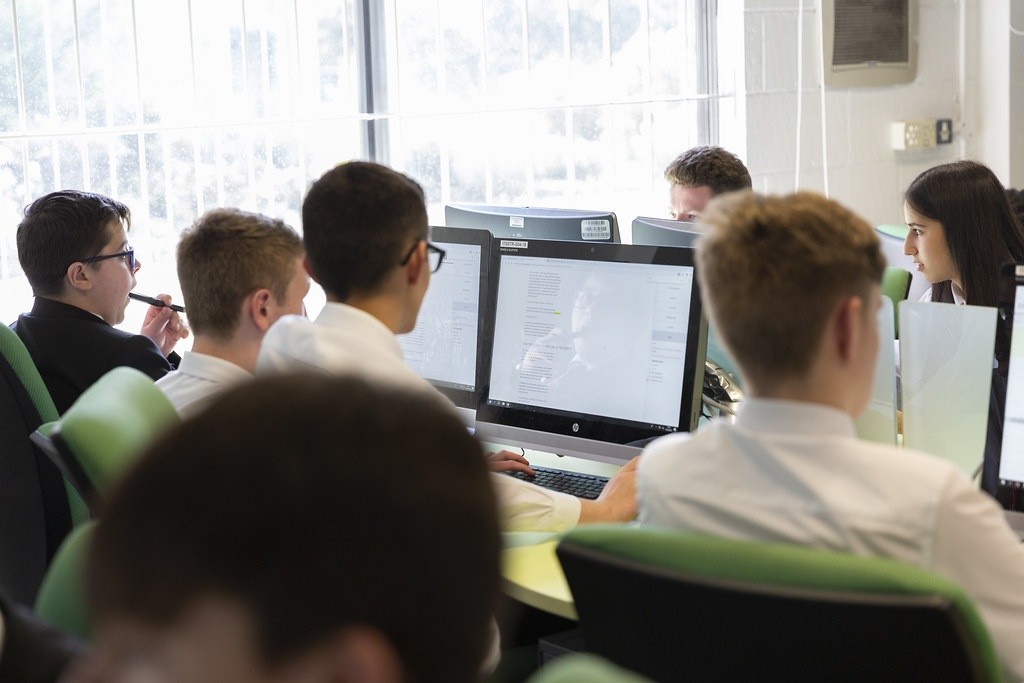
[498,465,611,500]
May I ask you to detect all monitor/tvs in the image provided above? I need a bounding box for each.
[394,205,747,465]
[980,260,1024,516]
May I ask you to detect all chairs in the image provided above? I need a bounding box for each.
[0,266,1006,683]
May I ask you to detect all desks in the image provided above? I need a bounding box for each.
[481,443,620,623]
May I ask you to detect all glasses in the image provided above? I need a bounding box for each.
[66,246,136,274]
[400,239,445,272]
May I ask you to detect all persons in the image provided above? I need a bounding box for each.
[258,161,639,549]
[510,265,658,422]
[150,208,310,422]
[664,145,753,223]
[7,190,190,418]
[633,189,1024,681]
[58,369,499,682]
[897,161,1024,435]
